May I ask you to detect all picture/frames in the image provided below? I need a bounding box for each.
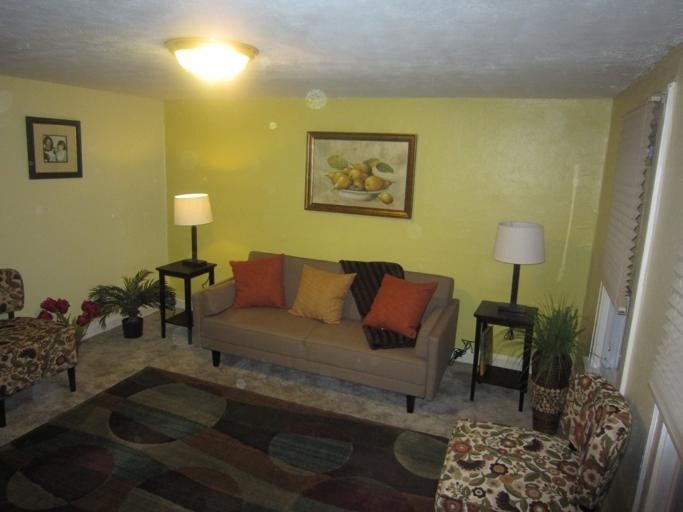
[25,116,84,178]
[303,131,417,220]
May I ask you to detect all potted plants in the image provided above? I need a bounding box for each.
[89,268,177,338]
[499,300,599,435]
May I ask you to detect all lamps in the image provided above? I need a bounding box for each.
[493,220,546,312]
[165,39,258,84]
[173,193,214,266]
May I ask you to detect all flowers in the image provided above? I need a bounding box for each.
[38,297,101,347]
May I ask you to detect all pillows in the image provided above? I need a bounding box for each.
[227,254,438,339]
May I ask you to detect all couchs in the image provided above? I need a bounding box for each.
[191,252,460,414]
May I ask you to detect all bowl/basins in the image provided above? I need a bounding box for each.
[331,180,392,202]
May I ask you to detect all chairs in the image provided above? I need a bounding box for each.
[435,372,632,512]
[0,268,78,427]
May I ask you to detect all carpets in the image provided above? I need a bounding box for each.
[0,366,450,512]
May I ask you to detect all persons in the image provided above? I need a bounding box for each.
[57,140,69,164]
[43,136,57,163]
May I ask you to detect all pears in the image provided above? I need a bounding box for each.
[326,162,393,204]
